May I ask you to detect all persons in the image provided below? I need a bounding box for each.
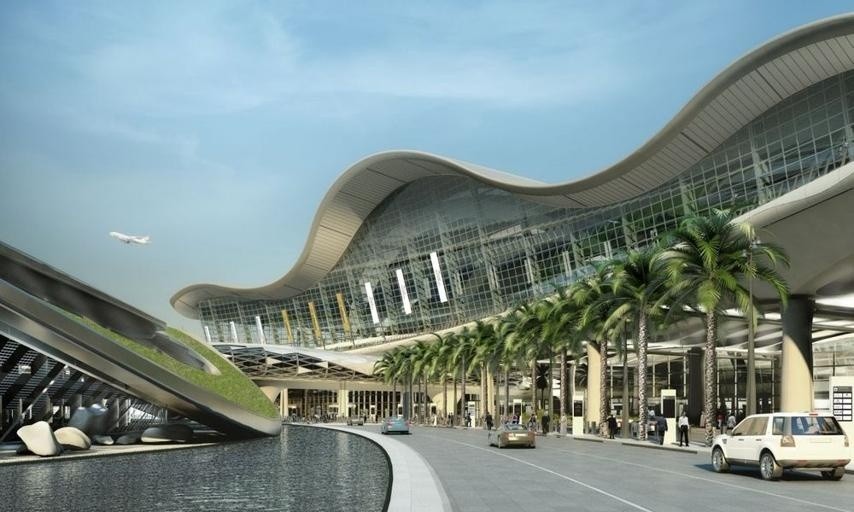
[485,412,492,430]
[530,414,537,431]
[542,411,550,434]
[448,412,453,426]
[679,411,689,446]
[466,413,472,427]
[656,414,668,444]
[513,411,521,424]
[604,414,616,439]
[717,411,725,427]
[728,412,736,428]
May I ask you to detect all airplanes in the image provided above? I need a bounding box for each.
[108,231,152,246]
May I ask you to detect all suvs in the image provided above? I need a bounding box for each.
[709,413,851,481]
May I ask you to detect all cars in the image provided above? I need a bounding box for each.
[488,423,536,449]
[380,416,410,435]
[347,415,363,426]
